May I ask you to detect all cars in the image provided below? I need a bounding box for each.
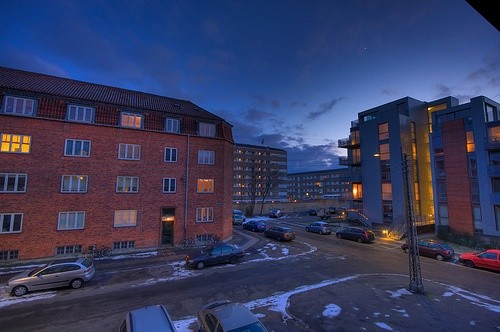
[242,220,267,233]
[197,299,269,332]
[185,242,245,270]
[401,238,455,261]
[232,209,246,226]
[460,248,500,272]
[336,227,376,243]
[268,209,287,218]
[305,222,331,235]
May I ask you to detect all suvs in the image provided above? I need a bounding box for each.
[119,304,178,332]
[264,225,296,242]
[4,256,96,296]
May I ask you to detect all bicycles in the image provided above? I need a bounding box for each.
[85,243,111,260]
[179,235,201,250]
